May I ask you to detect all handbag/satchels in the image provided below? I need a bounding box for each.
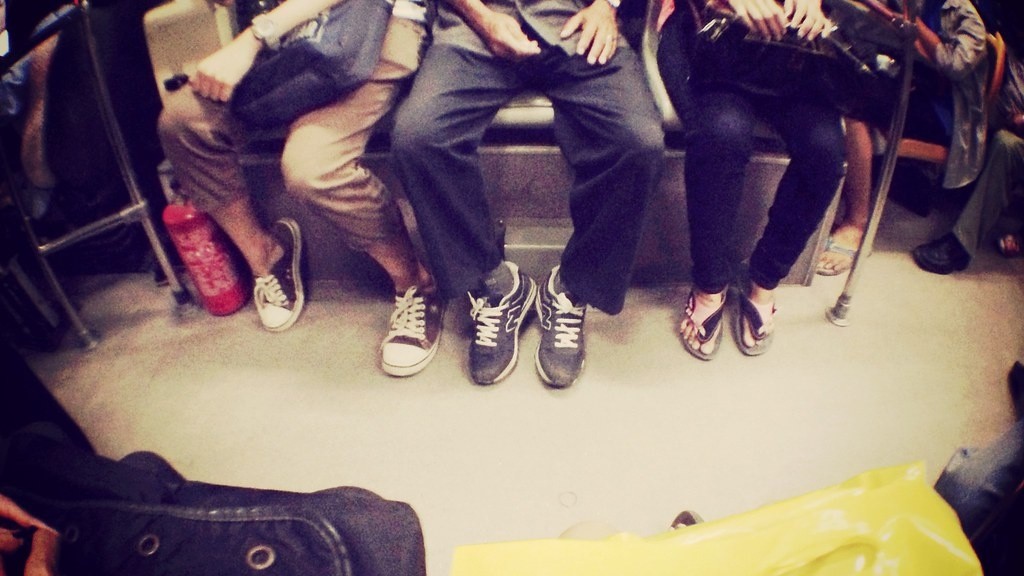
[53,452,427,576]
[686,7,876,107]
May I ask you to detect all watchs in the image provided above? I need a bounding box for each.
[252,14,279,49]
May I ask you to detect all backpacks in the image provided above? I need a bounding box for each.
[230,0,393,131]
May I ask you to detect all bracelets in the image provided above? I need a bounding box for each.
[608,0,620,7]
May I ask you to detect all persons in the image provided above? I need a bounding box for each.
[656,0,850,361]
[815,0,987,279]
[390,0,663,387]
[914,63,1024,272]
[159,0,444,376]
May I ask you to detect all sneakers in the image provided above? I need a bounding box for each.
[911,231,970,274]
[251,216,307,333]
[377,275,446,378]
[467,260,538,386]
[534,265,587,389]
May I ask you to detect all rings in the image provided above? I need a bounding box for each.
[607,36,613,41]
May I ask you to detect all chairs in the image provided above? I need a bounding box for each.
[878,31,1008,164]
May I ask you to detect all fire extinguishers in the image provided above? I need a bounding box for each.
[158,178,254,317]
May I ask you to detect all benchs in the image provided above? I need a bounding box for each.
[141,1,848,288]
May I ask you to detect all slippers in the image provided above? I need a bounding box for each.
[814,233,874,277]
[678,284,723,361]
[735,290,777,356]
